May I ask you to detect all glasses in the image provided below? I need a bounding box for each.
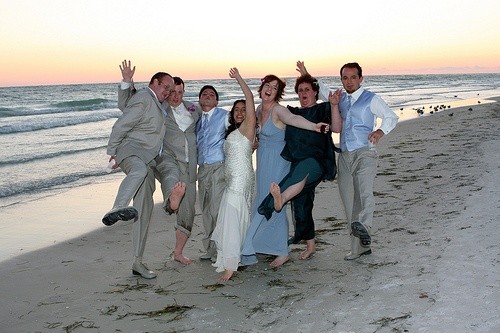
[160,82,173,92]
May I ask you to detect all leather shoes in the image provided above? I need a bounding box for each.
[132,256,155,279]
[102,205,138,226]
[351,220,373,248]
[344,247,372,260]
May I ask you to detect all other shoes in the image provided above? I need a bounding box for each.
[200,250,216,262]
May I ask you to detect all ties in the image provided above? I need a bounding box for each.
[201,113,206,128]
[348,96,351,109]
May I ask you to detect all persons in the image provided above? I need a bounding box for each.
[241,74,330,267]
[102,72,175,279]
[118,59,201,264]
[195,85,231,262]
[258,74,342,259]
[296,60,399,261]
[210,67,256,282]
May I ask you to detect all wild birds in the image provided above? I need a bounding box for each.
[399,94,481,117]
